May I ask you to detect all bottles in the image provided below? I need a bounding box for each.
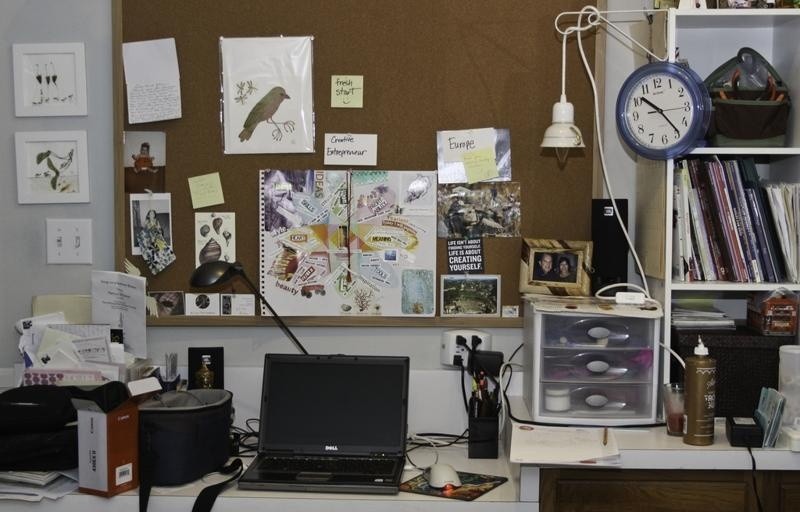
[195,353,216,390]
[683,343,719,447]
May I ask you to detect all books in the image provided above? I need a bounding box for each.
[760,387,787,447]
[0,270,149,507]
[671,154,800,282]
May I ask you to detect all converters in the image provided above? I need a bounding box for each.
[468,351,504,377]
[725,413,764,448]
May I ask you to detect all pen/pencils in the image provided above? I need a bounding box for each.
[468,370,502,418]
[603,427,608,446]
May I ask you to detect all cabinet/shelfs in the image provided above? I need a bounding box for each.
[633,8,799,417]
[525,297,662,425]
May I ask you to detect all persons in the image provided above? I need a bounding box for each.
[132,143,158,174]
[535,253,557,280]
[144,209,162,235]
[555,256,577,282]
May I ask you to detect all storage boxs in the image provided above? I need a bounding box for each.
[669,320,797,417]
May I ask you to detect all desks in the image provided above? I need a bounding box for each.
[508,394,799,511]
[0,436,522,512]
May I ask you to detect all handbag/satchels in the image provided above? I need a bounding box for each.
[701,45,794,149]
[134,383,244,512]
[0,380,131,473]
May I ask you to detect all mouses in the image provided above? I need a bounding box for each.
[421,463,462,490]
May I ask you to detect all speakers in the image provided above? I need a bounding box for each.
[591,198,628,298]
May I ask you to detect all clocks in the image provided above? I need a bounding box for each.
[615,61,714,164]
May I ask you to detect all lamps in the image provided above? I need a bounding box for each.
[538,29,589,164]
[188,260,310,354]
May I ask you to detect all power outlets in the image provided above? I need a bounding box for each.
[444,327,493,368]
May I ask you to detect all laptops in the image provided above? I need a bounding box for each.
[238,353,410,495]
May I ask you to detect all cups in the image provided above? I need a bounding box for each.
[543,386,571,413]
[661,382,683,438]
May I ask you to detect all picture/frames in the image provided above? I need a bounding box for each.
[11,40,91,118]
[13,129,92,205]
[517,235,594,297]
[437,272,502,320]
